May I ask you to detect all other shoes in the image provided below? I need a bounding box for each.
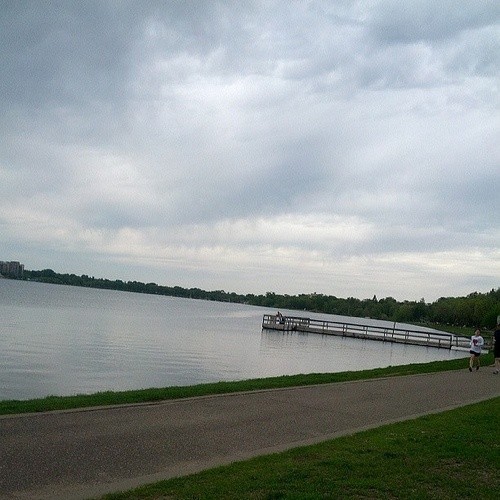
[469,367,472,372]
[476,366,480,370]
[493,370,499,374]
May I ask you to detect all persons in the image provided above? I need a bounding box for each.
[468,329,484,372]
[492,322,500,375]
[276,311,284,324]
